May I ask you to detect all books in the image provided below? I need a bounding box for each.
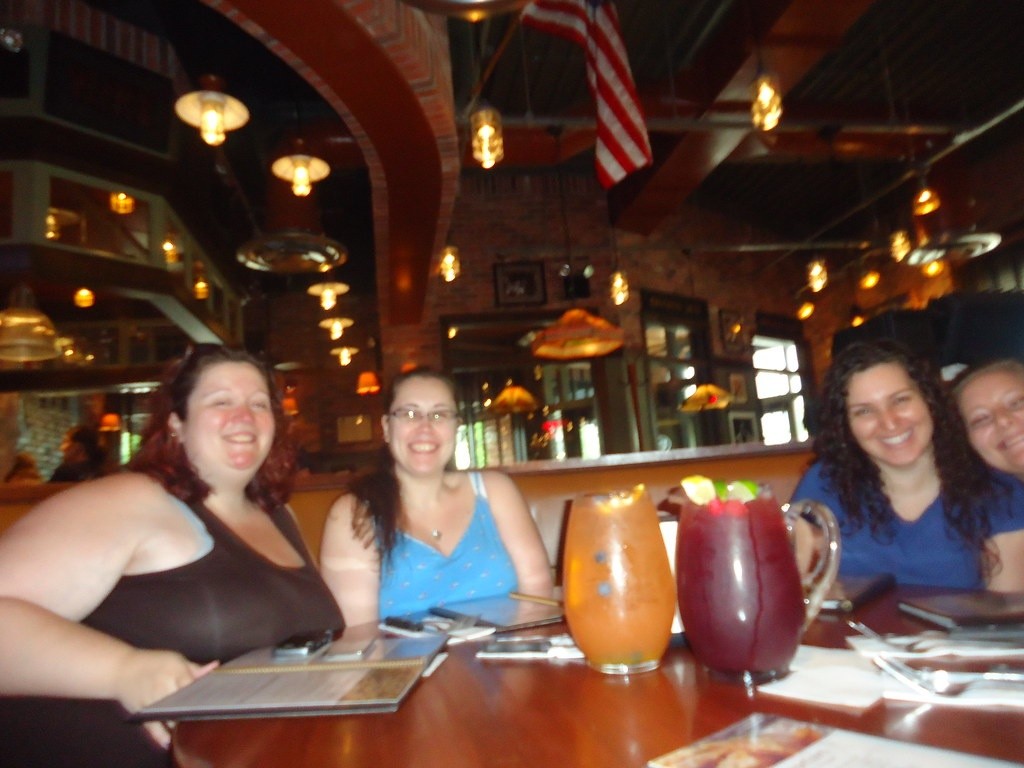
[450,362,595,468]
[124,590,1024,768]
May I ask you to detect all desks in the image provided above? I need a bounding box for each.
[168,572,1024,768]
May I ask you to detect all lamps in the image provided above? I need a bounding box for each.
[0,0,1022,443]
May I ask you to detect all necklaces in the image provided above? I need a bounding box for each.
[431,530,442,540]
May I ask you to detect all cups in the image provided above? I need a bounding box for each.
[674,480,842,690]
[560,489,682,676]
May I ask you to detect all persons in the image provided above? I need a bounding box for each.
[0,393,42,482]
[49,425,108,484]
[786,338,1024,593]
[951,357,1024,483]
[0,344,344,768]
[319,364,554,630]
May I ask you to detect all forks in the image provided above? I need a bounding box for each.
[847,621,1024,656]
[871,652,1024,696]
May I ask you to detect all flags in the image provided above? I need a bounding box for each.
[522,0,653,190]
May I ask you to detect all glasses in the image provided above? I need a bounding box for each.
[387,407,456,423]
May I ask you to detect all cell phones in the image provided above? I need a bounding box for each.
[273,634,329,660]
[821,573,890,611]
